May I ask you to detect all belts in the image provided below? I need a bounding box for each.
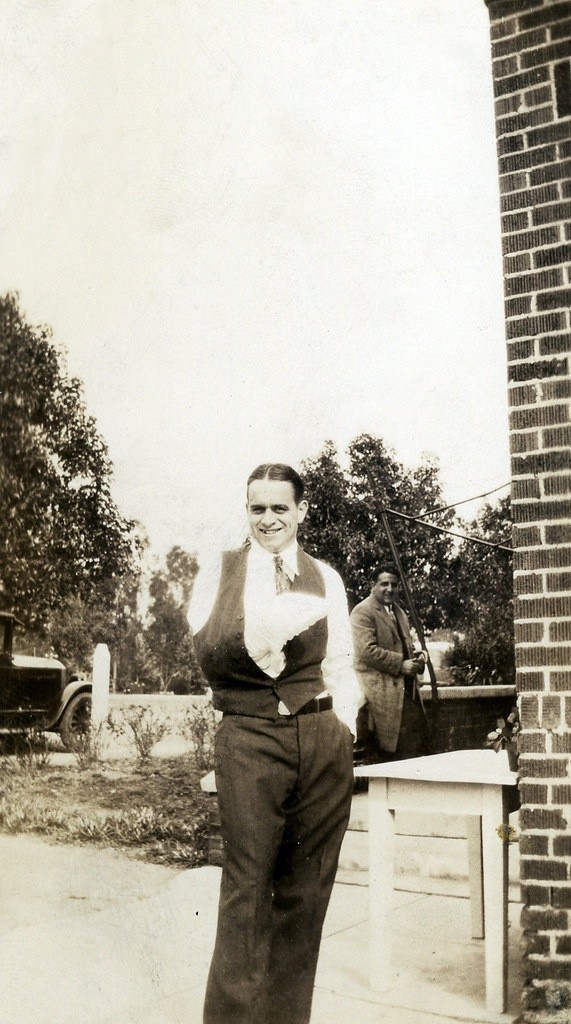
[295,696,333,715]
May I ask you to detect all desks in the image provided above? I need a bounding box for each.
[353,747,518,1014]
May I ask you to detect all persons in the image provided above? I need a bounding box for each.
[186,462,365,1024]
[348,561,437,764]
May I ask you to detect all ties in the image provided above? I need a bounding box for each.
[273,556,290,595]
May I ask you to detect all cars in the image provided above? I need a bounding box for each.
[0,610,95,752]
